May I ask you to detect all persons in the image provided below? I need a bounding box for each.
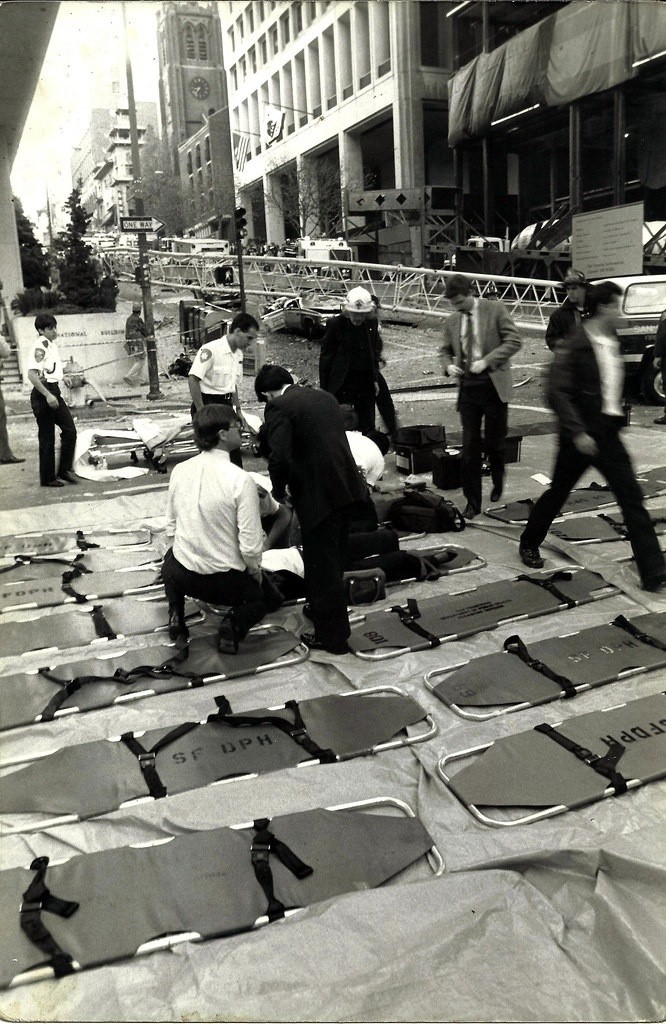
[652,309,666,425]
[545,268,595,353]
[161,403,283,654]
[244,470,457,596]
[439,274,522,520]
[0,337,25,467]
[253,364,373,655]
[230,239,296,272]
[123,303,151,387]
[27,314,79,487]
[345,429,390,496]
[187,313,259,470]
[319,287,398,434]
[519,280,666,592]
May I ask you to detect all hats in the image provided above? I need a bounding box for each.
[133,303,141,311]
[558,271,590,286]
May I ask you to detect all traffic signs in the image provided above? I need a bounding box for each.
[119,217,165,232]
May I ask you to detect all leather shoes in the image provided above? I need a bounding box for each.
[218,617,238,655]
[302,604,316,622]
[643,578,666,590]
[169,615,189,639]
[300,633,327,650]
[653,415,666,424]
[519,543,543,569]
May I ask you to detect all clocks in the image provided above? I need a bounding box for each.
[189,76,210,99]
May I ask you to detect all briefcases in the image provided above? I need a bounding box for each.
[394,425,446,445]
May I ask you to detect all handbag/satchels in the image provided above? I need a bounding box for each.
[342,567,386,606]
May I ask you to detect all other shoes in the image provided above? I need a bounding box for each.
[490,487,502,502]
[461,502,481,519]
[47,480,64,486]
[0,456,25,464]
[57,469,80,483]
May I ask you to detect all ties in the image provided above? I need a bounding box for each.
[463,311,475,382]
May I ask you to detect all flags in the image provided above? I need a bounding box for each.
[260,105,285,147]
[232,134,250,172]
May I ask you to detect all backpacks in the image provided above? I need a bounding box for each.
[385,491,466,533]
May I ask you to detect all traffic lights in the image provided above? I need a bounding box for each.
[233,206,248,240]
[215,267,234,284]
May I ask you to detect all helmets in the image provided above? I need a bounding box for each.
[340,285,377,313]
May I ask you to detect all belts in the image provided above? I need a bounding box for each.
[221,394,233,399]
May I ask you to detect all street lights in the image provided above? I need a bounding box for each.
[45,147,82,244]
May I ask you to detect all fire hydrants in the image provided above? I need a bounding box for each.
[62,355,88,410]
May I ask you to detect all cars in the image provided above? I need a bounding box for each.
[591,273,666,406]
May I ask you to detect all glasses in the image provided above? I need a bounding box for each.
[219,421,241,431]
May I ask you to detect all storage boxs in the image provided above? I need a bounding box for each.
[395,425,447,475]
[482,433,523,463]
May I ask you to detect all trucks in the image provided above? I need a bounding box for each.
[444,235,512,268]
[296,237,354,281]
[170,237,231,264]
